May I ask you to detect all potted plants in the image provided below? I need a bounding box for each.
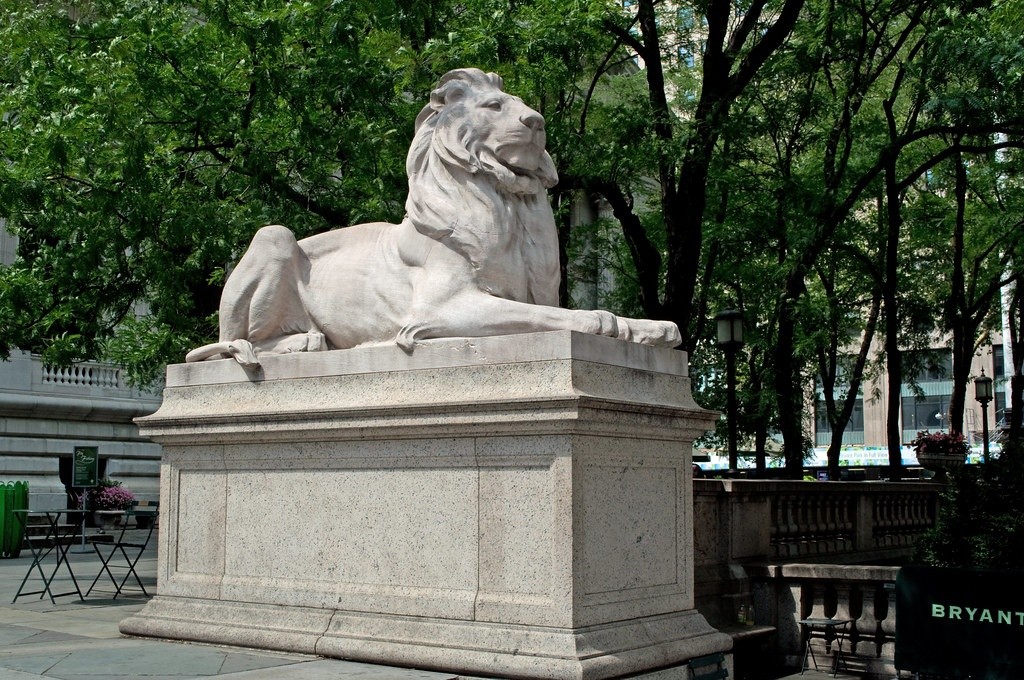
[98,478,123,530]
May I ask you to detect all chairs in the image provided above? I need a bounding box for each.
[85,505,158,600]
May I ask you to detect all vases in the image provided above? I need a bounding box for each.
[916,452,967,483]
[93,512,116,530]
[135,512,157,530]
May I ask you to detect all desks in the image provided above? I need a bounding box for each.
[95,509,157,517]
[797,618,854,678]
[11,509,90,604]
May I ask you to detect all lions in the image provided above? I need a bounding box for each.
[184,68,682,367]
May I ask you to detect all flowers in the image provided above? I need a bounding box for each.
[902,428,972,455]
[94,486,134,510]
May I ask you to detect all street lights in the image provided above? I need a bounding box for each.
[974,365,994,463]
[715,306,744,478]
